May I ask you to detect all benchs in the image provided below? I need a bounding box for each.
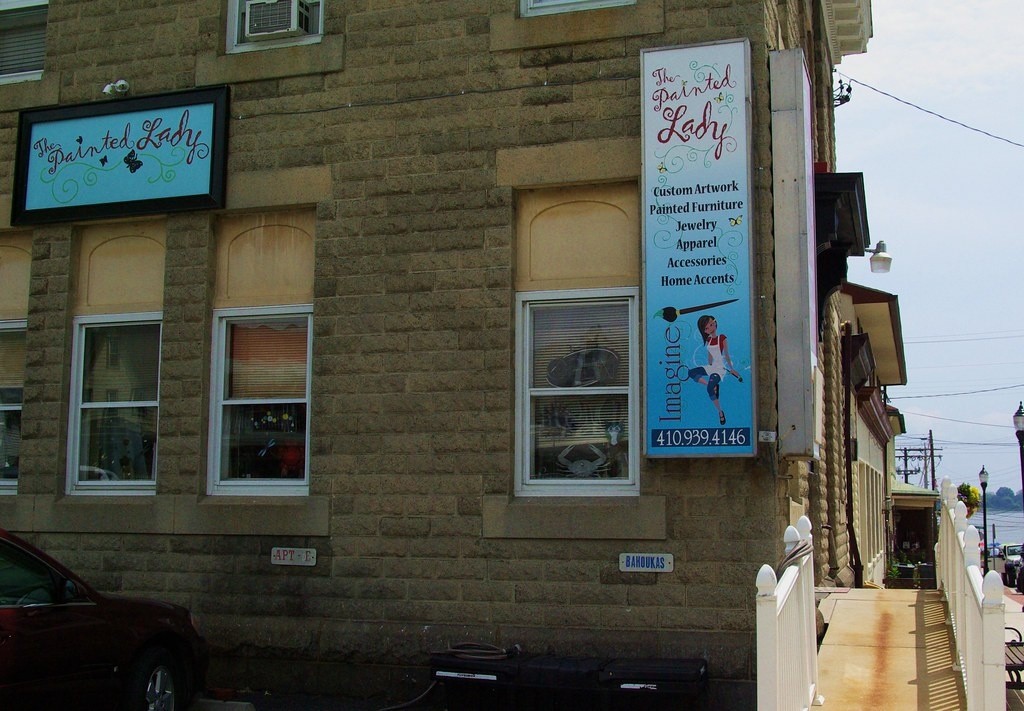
[1005,627,1024,690]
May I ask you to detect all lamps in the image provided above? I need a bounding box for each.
[864,240,892,273]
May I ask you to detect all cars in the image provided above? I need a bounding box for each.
[0,526,208,711]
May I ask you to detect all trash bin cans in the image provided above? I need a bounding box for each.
[427,645,710,711]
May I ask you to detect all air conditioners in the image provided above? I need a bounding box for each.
[245,0,313,41]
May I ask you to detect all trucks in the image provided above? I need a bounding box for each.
[988,544,1024,587]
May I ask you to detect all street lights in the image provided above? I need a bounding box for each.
[978,465,989,576]
[1013,401,1024,513]
